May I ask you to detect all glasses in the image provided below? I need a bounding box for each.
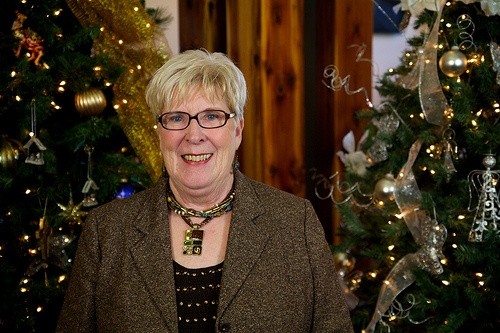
[157,110,236,127]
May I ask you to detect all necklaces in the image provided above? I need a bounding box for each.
[166,180,235,255]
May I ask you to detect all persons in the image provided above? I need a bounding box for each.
[57,47,353,332]
